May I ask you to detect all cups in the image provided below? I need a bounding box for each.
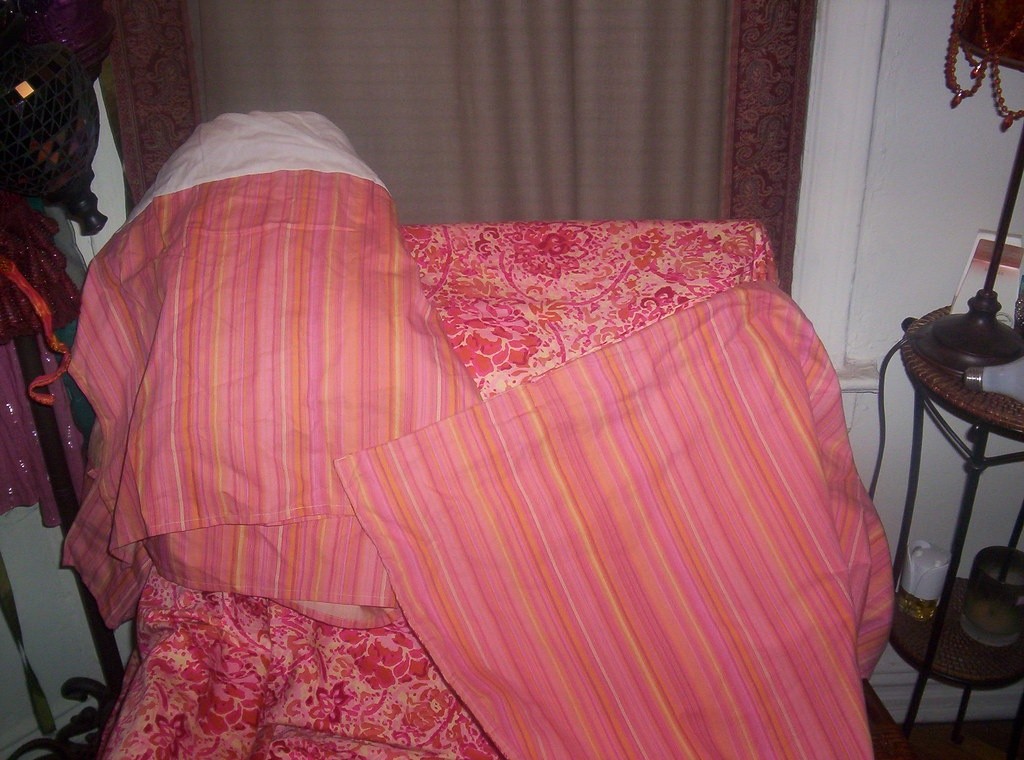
[961,546,1024,648]
[894,539,957,622]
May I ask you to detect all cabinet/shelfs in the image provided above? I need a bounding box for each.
[862,304,1024,758]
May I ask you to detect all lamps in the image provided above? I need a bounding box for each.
[913,0,1024,376]
